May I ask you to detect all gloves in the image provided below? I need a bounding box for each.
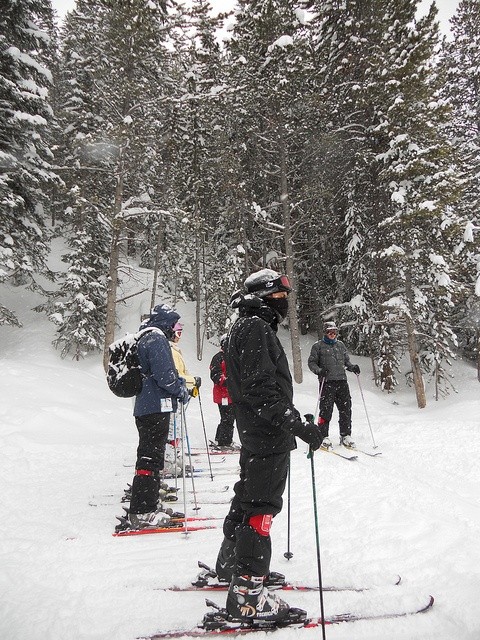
[320,367,329,376]
[292,413,323,449]
[189,387,198,397]
[194,376,202,387]
[176,388,189,404]
[348,364,360,374]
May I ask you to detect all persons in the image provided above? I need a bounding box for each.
[139,319,169,500]
[307,321,360,450]
[161,322,201,477]
[128,303,189,529]
[210,337,242,451]
[216,268,322,624]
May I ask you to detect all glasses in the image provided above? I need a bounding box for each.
[244,275,292,293]
[174,331,182,339]
[325,331,337,335]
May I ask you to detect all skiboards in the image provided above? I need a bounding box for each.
[65,516,225,540]
[133,574,434,640]
[177,446,243,456]
[115,461,242,478]
[90,486,235,507]
[318,439,382,461]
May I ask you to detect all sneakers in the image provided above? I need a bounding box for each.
[221,442,242,450]
[165,459,183,468]
[216,537,270,582]
[129,511,171,527]
[226,574,289,624]
[159,489,167,501]
[321,436,332,449]
[163,461,181,474]
[159,482,168,490]
[340,434,355,447]
[156,504,173,515]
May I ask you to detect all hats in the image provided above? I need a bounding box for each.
[244,269,290,297]
[172,322,182,331]
[323,322,338,330]
[220,333,227,346]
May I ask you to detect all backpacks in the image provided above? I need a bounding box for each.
[107,327,161,397]
